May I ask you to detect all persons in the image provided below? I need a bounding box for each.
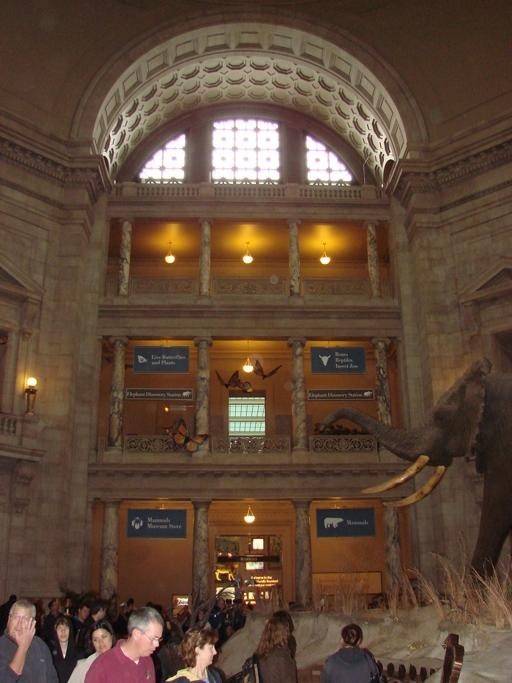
[317,622,382,682]
[249,609,303,681]
[2,590,257,683]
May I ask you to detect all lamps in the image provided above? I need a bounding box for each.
[164,239,177,264]
[243,503,256,525]
[240,351,255,373]
[318,241,333,265]
[241,240,255,264]
[25,375,38,413]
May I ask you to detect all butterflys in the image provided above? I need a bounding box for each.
[170,417,207,451]
[215,368,240,389]
[252,360,282,379]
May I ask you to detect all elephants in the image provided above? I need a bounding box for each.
[316,356,511,603]
